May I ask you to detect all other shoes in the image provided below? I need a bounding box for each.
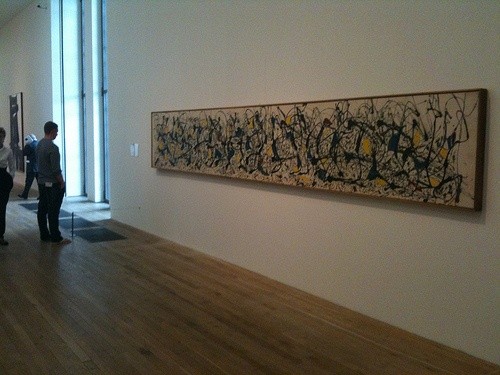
[59,238,72,245]
[0,236,8,246]
[17,192,29,200]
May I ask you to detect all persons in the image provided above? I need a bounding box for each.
[0,127,16,245]
[18,133,39,200]
[36,121,72,245]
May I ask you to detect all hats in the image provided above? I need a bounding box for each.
[24,133,38,145]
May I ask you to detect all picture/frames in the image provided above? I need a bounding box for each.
[151,88,488,212]
